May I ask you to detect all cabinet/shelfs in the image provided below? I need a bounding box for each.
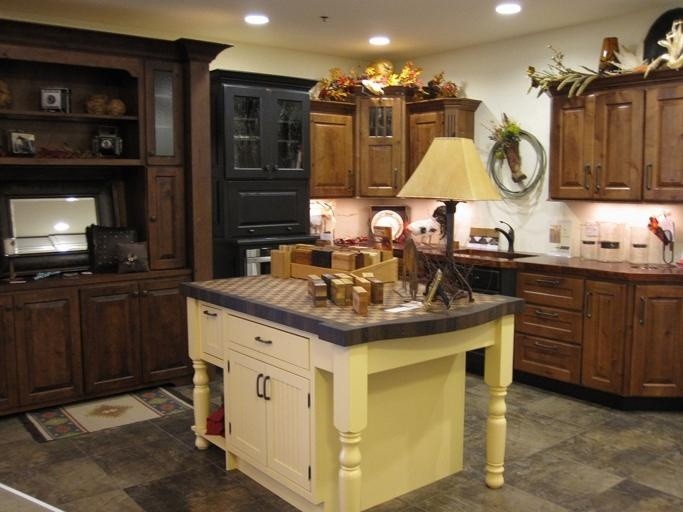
[643,68,683,204]
[206,68,317,182]
[1,286,84,416]
[310,98,354,197]
[512,266,584,389]
[582,279,629,398]
[546,71,643,202]
[79,276,194,403]
[177,274,526,512]
[404,97,481,180]
[0,18,234,291]
[212,179,311,240]
[351,86,403,201]
[223,237,320,280]
[627,282,683,400]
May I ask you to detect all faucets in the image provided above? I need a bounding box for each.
[494,220,515,253]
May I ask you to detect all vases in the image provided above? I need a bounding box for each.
[598,33,622,75]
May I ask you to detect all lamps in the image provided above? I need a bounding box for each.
[392,135,506,312]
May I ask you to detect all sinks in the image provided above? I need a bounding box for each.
[453,248,540,266]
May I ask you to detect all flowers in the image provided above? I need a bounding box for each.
[521,22,683,98]
[480,109,527,185]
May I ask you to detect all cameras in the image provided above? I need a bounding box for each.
[40,87,71,113]
[92,134,124,159]
[8,131,35,155]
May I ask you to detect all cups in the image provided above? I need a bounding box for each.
[578,220,650,266]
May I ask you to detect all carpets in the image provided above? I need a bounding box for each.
[18,384,193,442]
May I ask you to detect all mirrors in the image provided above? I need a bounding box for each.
[0,180,126,277]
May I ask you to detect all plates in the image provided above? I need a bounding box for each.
[642,6,683,67]
[370,210,403,243]
